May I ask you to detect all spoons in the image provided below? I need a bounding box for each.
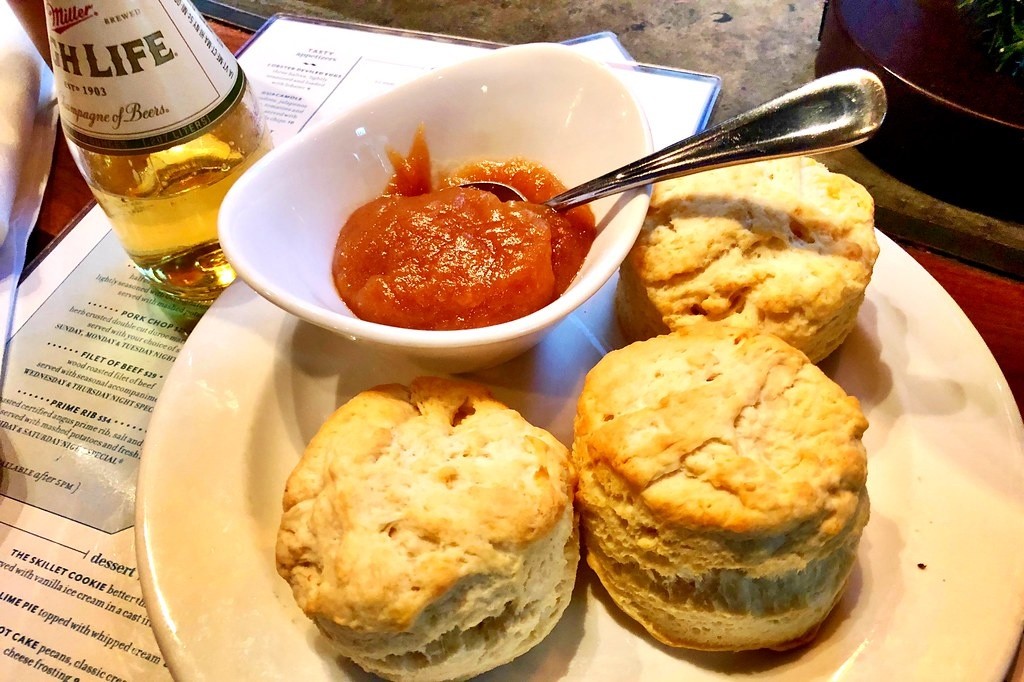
[452,70,887,210]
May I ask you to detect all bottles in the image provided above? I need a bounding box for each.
[43,0,277,311]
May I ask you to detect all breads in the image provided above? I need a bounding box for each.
[569,323,870,653]
[274,377,582,682]
[615,156,879,367]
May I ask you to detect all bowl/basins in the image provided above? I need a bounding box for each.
[218,39,653,373]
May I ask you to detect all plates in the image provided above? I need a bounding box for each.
[136,228,1023,682]
